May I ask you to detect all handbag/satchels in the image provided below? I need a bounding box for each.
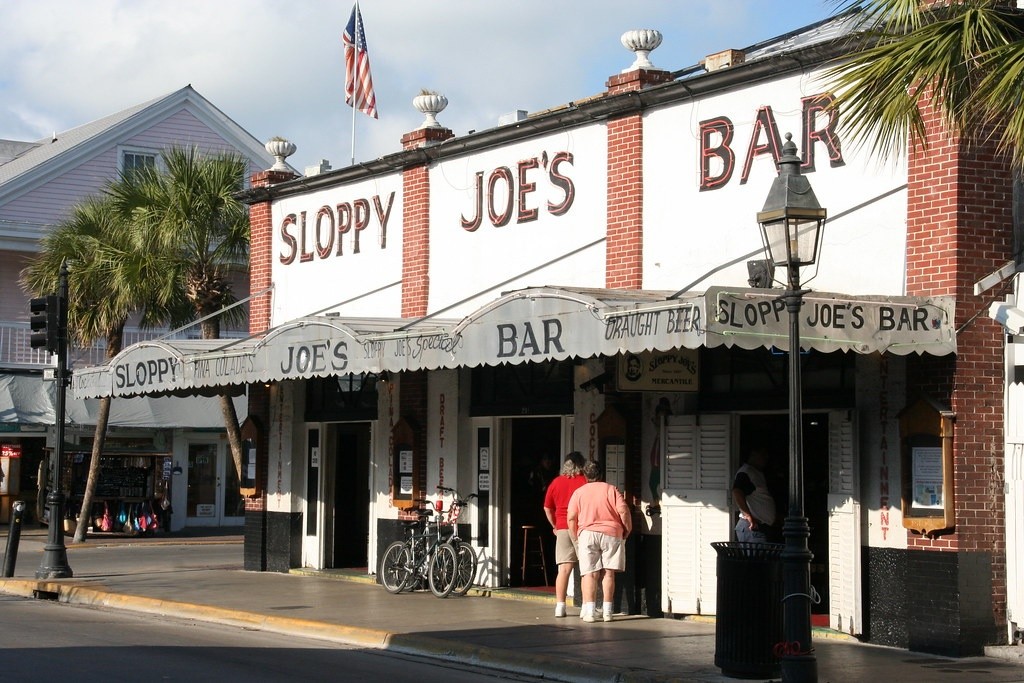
[101,499,159,534]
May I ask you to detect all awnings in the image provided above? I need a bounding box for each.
[0,369,248,428]
[73,180,957,401]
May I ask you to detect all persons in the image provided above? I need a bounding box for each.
[733,438,776,543]
[625,355,641,381]
[543,453,588,614]
[567,459,631,622]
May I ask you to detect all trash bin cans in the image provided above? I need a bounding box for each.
[710,539,819,683]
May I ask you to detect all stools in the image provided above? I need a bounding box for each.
[520,526,548,586]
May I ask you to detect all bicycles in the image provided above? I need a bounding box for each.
[379,486,479,599]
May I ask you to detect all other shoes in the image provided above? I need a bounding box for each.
[554,606,566,617]
[583,610,595,623]
[580,610,585,618]
[603,612,613,622]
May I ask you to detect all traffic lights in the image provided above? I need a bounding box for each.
[29,297,47,348]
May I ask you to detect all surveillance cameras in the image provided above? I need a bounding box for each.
[989,301,1024,336]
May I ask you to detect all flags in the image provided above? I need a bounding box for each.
[342,0,378,119]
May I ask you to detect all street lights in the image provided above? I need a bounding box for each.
[754,133,828,683]
